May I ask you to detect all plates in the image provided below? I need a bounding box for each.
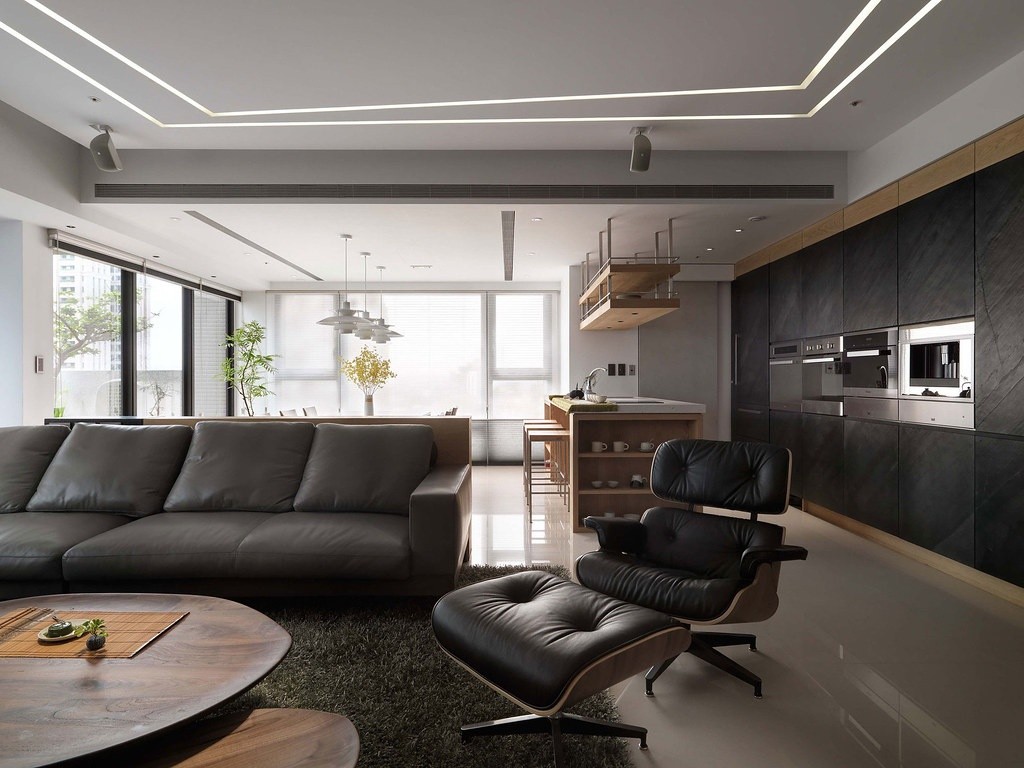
[38,619,91,641]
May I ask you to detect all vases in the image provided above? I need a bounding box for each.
[364,394,373,416]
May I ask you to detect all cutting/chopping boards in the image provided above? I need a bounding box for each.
[551,399,617,412]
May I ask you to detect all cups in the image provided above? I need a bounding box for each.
[591,442,607,452]
[641,442,655,451]
[613,441,629,452]
[603,512,615,517]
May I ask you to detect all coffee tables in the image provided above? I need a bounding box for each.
[0,592,360,768]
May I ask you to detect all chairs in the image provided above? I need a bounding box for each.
[445,408,458,415]
[574,438,809,699]
[280,407,318,417]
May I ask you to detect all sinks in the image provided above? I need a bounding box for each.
[605,396,664,404]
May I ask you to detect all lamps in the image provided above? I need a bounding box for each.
[629,126,652,174]
[89,124,125,173]
[317,234,403,344]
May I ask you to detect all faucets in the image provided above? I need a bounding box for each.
[584,367,608,399]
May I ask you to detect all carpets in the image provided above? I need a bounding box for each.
[198,567,636,768]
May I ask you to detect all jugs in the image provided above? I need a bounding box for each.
[630,476,648,489]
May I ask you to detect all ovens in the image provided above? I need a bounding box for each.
[769,316,975,429]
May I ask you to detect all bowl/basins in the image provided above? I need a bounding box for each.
[592,480,603,488]
[607,481,619,487]
[587,393,607,402]
[624,513,640,521]
[616,294,642,298]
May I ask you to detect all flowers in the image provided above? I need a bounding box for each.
[338,344,397,395]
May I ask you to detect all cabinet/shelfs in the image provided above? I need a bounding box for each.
[729,149,1024,610]
[569,413,704,534]
[578,217,680,332]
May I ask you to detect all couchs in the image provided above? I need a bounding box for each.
[0,415,473,599]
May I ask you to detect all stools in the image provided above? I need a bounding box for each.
[430,570,693,768]
[522,419,570,523]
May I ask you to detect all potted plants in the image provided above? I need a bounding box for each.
[74,618,107,651]
